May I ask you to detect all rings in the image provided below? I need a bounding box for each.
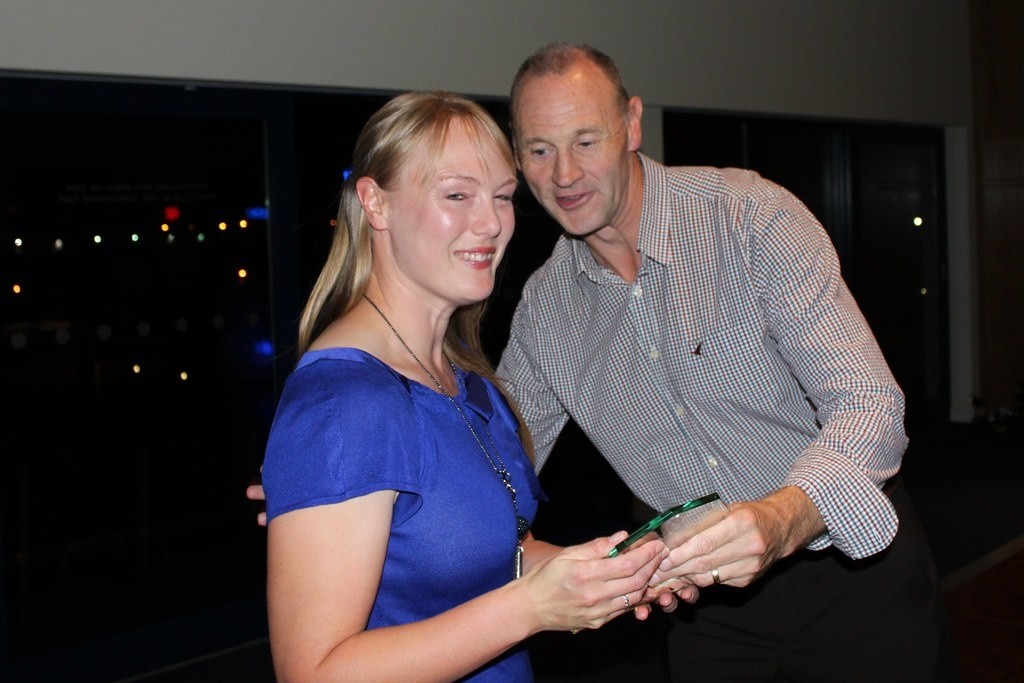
[623,595,629,610]
[711,568,720,584]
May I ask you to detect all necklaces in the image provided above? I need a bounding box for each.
[362,292,528,580]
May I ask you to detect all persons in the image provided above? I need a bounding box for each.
[246,41,962,683]
[259,90,698,683]
